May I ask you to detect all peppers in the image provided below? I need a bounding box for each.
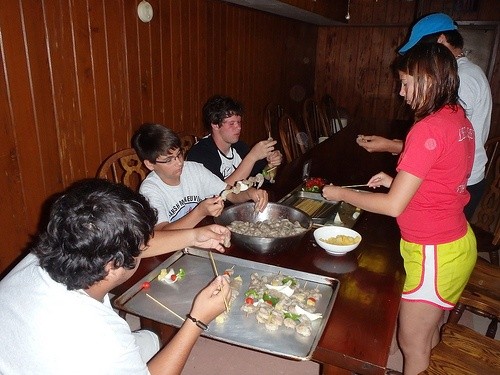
[262,170,268,176]
[244,277,301,319]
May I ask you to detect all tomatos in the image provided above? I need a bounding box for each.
[305,177,325,187]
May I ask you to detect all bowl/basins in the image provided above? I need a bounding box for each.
[314,225,362,255]
[214,201,313,255]
[312,251,359,274]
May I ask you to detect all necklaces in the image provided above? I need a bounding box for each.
[455,52,466,62]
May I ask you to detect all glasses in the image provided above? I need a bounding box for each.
[155,148,186,165]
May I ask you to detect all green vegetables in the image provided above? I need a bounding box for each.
[302,186,323,193]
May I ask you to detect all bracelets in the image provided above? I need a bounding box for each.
[187,314,209,332]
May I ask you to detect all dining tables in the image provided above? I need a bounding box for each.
[108,120,413,375]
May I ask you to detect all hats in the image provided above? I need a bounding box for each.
[399,13,458,55]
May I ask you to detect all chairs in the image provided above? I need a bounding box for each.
[99,94,500,375]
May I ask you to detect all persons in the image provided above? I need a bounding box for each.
[0,178,234,375]
[321,43,478,375]
[353,12,494,219]
[184,96,283,187]
[129,124,269,230]
